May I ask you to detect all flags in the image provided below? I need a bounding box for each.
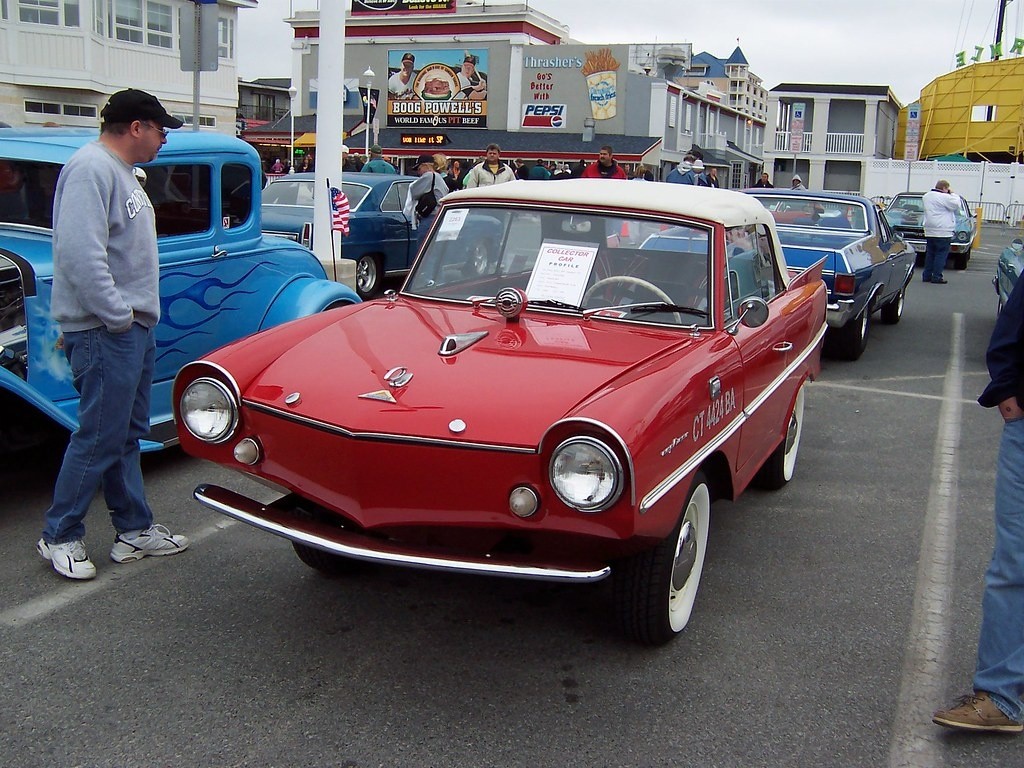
[329,186,350,236]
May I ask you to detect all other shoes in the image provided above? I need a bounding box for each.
[923,278,949,284]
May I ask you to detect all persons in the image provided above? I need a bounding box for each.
[581,145,627,179]
[360,145,396,173]
[432,153,453,193]
[388,53,420,100]
[789,174,806,191]
[707,168,719,188]
[35,88,190,579]
[260,144,315,192]
[510,158,586,179]
[930,269,1024,732]
[409,153,449,254]
[466,142,517,189]
[922,179,961,284]
[444,155,483,194]
[362,93,376,110]
[384,157,398,170]
[342,144,368,173]
[455,55,488,100]
[625,164,653,182]
[666,155,720,188]
[751,173,774,188]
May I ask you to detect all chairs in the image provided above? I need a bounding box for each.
[146,172,192,234]
[793,217,852,229]
[903,205,919,212]
[611,260,707,324]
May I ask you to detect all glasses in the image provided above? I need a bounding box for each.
[147,123,170,139]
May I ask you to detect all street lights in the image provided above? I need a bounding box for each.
[363,66,376,164]
[288,86,298,175]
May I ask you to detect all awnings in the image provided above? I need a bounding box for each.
[343,128,664,165]
[692,144,733,167]
[292,133,347,147]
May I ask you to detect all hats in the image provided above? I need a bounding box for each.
[412,154,434,171]
[537,159,544,163]
[371,144,382,154]
[401,52,415,64]
[692,160,705,169]
[342,144,349,154]
[101,88,184,129]
[463,55,476,67]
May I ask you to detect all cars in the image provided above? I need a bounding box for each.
[170,176,830,641]
[883,191,978,270]
[261,172,504,301]
[638,187,918,358]
[0,129,356,454]
[992,237,1024,317]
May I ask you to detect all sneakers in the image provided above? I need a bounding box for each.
[35,536,98,581]
[933,691,1024,733]
[110,524,189,564]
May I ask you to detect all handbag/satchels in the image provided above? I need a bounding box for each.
[416,173,437,216]
